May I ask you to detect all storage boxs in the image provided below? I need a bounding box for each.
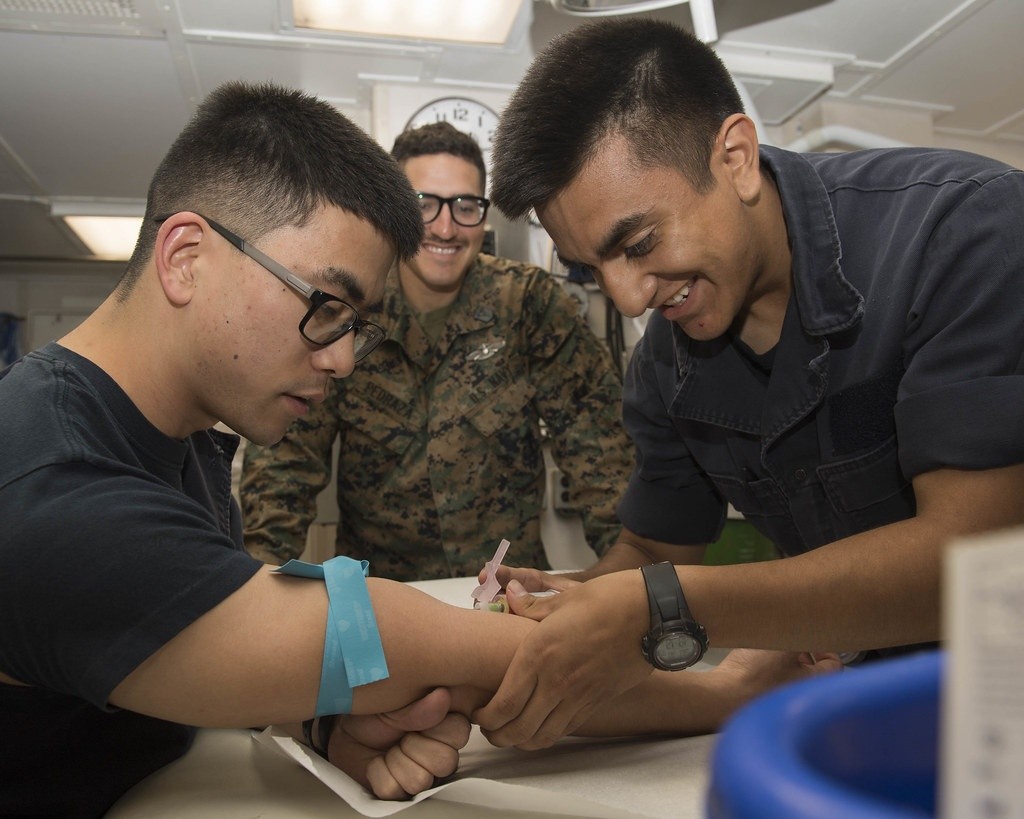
[936,523,1024,819]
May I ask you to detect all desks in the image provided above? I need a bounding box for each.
[106,568,859,819]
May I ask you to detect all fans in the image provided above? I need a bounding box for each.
[548,0,836,46]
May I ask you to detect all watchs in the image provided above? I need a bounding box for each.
[641,560,707,672]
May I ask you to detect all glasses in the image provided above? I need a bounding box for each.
[416,193,491,227]
[150,208,386,365]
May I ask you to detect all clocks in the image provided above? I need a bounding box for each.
[402,96,504,203]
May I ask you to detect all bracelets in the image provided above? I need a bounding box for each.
[302,710,341,761]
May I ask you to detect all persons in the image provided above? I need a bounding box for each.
[470,18,1024,752]
[1,82,847,819]
[238,121,639,585]
